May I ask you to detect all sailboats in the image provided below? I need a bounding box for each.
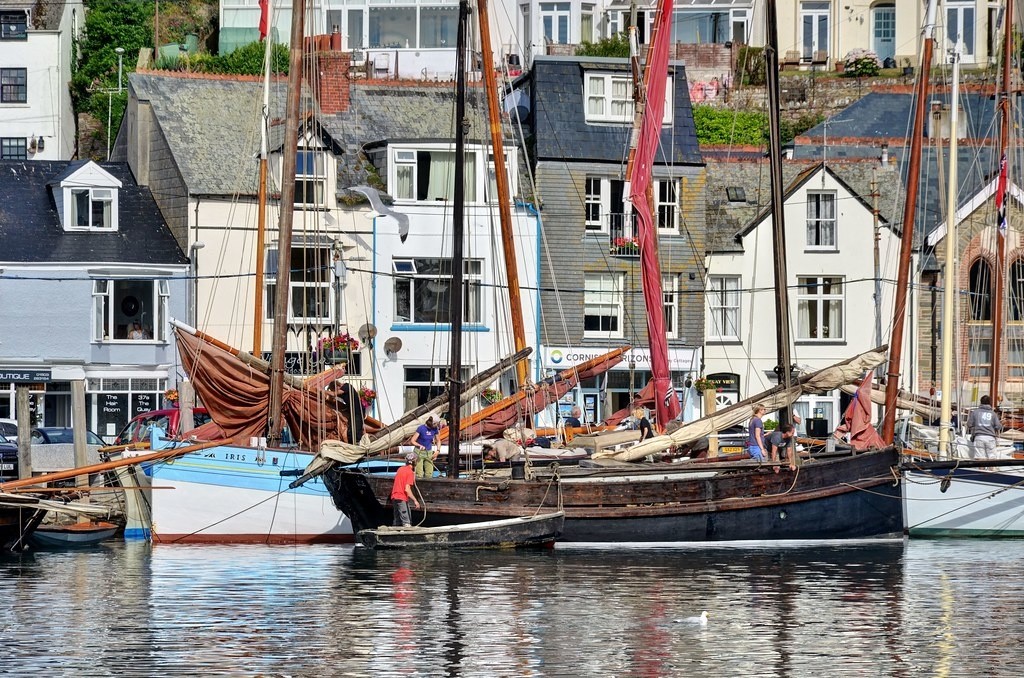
[3,0,1024,557]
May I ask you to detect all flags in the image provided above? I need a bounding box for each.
[258,0,267,43]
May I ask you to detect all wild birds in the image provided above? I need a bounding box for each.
[672,611,710,623]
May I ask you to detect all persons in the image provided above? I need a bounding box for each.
[127,318,151,340]
[410,417,442,478]
[390,454,421,525]
[666,420,710,462]
[564,404,583,427]
[967,395,1003,472]
[633,408,655,464]
[764,423,797,474]
[480,439,521,462]
[747,403,768,463]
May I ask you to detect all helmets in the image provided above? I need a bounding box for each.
[405,452,419,465]
